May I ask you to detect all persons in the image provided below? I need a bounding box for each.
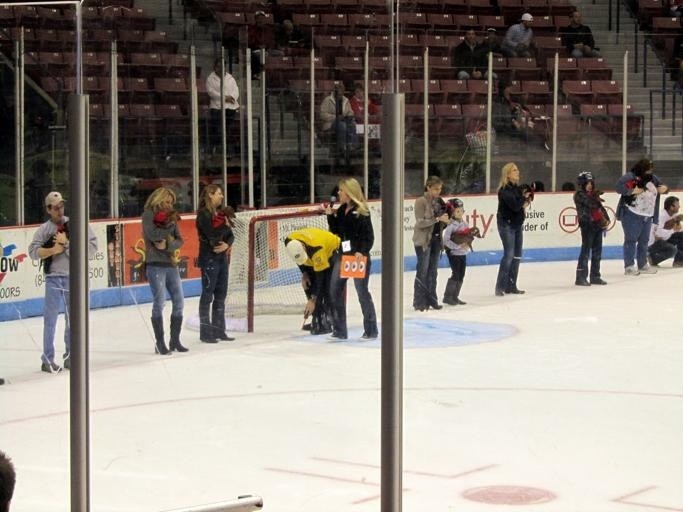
[247,1,311,80]
[283,227,341,335]
[659,0,682,22]
[324,177,379,341]
[28,160,67,208]
[450,13,546,154]
[646,196,682,267]
[495,163,535,296]
[205,58,240,151]
[614,158,658,276]
[573,171,611,286]
[411,174,473,311]
[27,191,97,372]
[140,187,190,356]
[193,184,234,342]
[560,11,599,57]
[319,81,360,158]
[350,84,383,155]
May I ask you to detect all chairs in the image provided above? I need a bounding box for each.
[627,0,682,89]
[0,0,247,140]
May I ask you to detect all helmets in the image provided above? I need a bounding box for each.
[578,171,593,184]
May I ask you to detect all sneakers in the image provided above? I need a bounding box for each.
[151,316,378,354]
[40,362,62,373]
[415,256,682,309]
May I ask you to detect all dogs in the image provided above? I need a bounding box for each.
[444,226,482,253]
[311,292,332,331]
[517,183,534,211]
[39,222,70,275]
[153,208,181,267]
[434,198,461,242]
[205,206,236,257]
[622,173,655,208]
[664,214,683,231]
[583,189,605,226]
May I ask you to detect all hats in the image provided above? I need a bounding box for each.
[521,14,535,23]
[45,192,66,210]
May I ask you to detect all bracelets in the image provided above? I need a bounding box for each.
[435,217,439,223]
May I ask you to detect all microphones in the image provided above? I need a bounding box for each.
[330,196,336,208]
